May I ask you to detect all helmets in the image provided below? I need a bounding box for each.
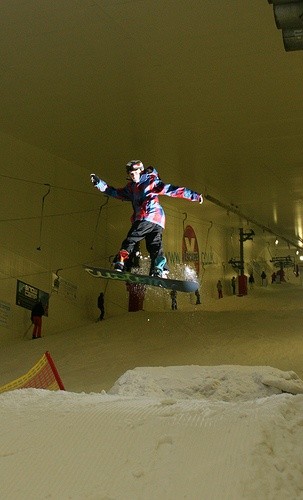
[126,161,145,173]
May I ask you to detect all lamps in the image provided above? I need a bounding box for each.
[296,246,300,255]
[274,234,279,245]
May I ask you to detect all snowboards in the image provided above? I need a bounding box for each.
[81,263,200,293]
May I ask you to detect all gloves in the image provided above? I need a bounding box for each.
[90,173,100,185]
[199,195,204,205]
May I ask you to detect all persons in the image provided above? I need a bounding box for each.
[195,289,201,304]
[31,300,45,340]
[249,274,254,290]
[170,290,177,310]
[261,271,266,283]
[272,272,276,283]
[98,292,104,320]
[232,277,237,292]
[217,280,223,298]
[90,160,204,276]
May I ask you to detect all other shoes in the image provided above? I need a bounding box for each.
[152,270,161,277]
[112,262,123,272]
